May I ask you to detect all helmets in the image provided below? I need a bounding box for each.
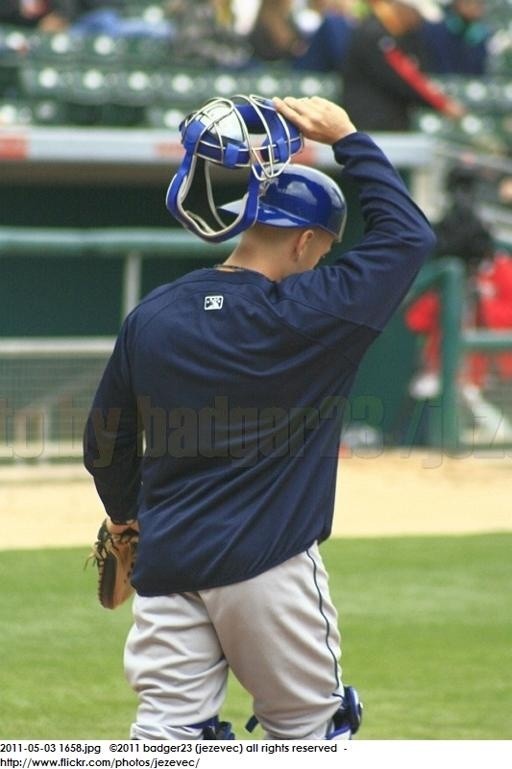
[166,95,346,244]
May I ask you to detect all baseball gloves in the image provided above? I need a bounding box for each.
[84,519,140,609]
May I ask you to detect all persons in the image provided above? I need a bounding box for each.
[430,168,493,274]
[81,95,439,742]
[0,1,510,80]
[404,283,490,400]
[340,3,464,132]
[494,172,511,206]
